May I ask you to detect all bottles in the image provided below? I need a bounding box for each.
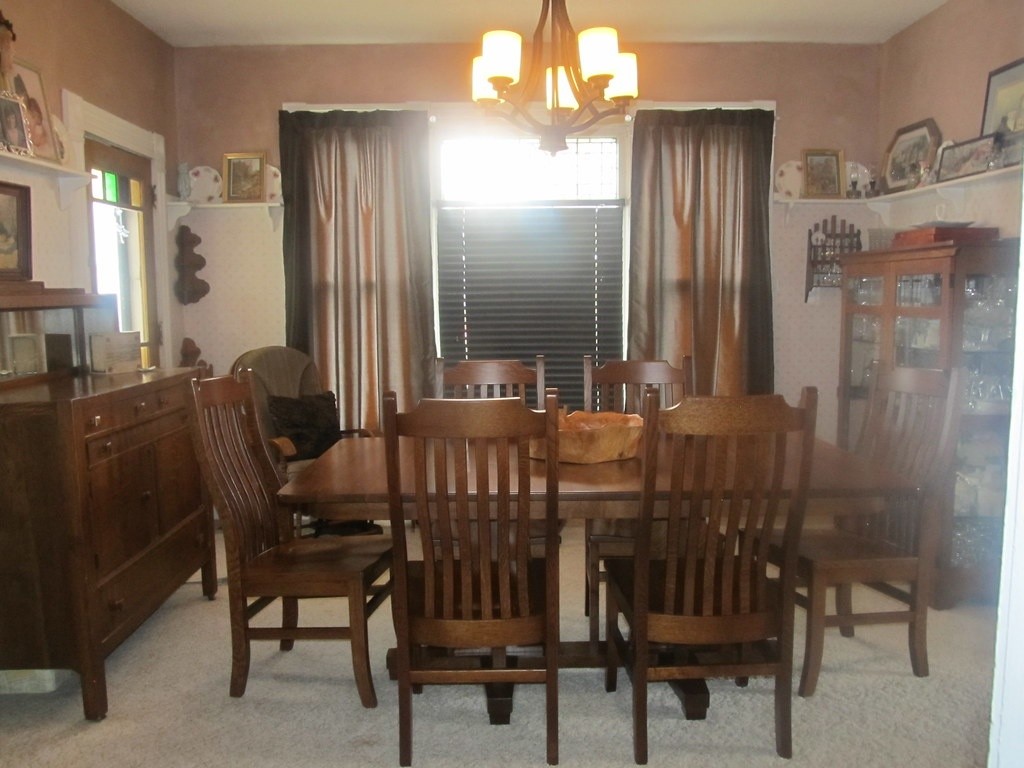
[987,115,1010,170]
[897,277,938,306]
[894,315,906,345]
[857,278,882,308]
[857,314,882,343]
[948,531,967,566]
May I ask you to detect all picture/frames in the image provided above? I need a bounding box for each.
[800,147,848,200]
[935,129,1006,182]
[0,91,37,162]
[880,118,943,194]
[4,57,63,166]
[222,150,267,204]
[979,57,1024,166]
[0,179,33,280]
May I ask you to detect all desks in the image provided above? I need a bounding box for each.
[276,417,933,726]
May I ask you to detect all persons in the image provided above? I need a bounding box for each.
[1,104,26,154]
[14,73,49,147]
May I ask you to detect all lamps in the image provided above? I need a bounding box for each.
[471,0,638,156]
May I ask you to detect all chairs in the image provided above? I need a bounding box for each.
[603,386,818,765]
[183,364,392,710]
[411,355,567,558]
[230,345,385,538]
[583,354,727,616]
[369,387,564,768]
[734,360,970,697]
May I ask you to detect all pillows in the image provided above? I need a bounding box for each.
[264,391,342,462]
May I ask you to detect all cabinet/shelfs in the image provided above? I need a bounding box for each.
[832,234,1020,610]
[0,364,218,722]
